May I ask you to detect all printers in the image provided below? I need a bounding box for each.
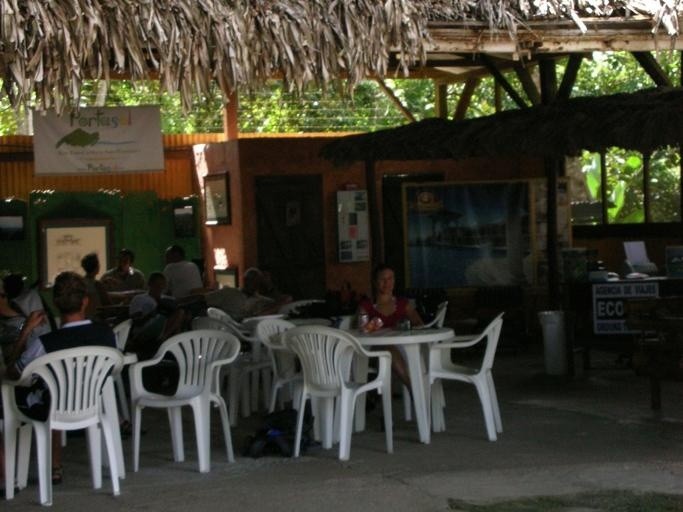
[617,259,659,278]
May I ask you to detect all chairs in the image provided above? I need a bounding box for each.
[425,313,504,442]
[2,347,126,507]
[102,296,448,425]
[280,325,392,461]
[0,293,506,507]
[127,331,235,473]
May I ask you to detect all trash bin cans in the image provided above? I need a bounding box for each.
[539,311,583,382]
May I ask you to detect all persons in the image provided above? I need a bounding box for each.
[356,264,424,430]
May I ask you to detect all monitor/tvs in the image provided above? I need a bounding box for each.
[664,246,683,277]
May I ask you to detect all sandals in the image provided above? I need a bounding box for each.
[51,461,65,485]
[120,419,148,440]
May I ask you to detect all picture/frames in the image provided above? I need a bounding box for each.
[37,216,113,292]
[204,170,231,227]
[213,265,239,291]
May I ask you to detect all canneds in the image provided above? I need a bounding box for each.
[359,313,369,331]
[395,319,411,331]
